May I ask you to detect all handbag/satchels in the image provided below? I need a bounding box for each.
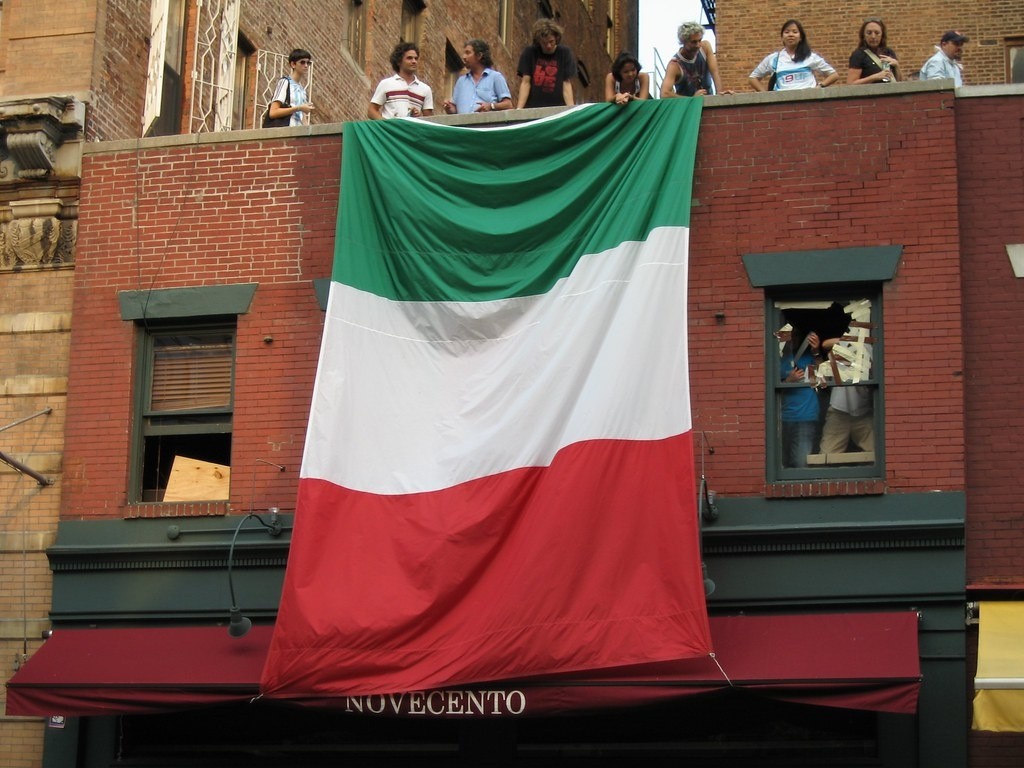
[262,77,291,128]
[768,51,779,91]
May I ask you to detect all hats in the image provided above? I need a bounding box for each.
[941,31,969,43]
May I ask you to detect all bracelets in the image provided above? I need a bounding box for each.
[491,103,495,110]
[812,353,820,356]
[818,83,824,88]
[298,105,300,111]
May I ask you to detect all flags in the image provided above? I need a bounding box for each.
[256,93,714,697]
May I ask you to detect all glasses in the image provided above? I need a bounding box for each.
[296,60,312,66]
[864,18,881,23]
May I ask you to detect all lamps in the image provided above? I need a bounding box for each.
[228,513,281,639]
[699,478,719,597]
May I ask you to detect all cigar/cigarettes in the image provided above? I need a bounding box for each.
[444,104,447,107]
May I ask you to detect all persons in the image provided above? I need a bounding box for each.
[846,19,903,84]
[605,51,649,104]
[516,18,577,109]
[781,331,824,468]
[442,38,513,115]
[269,49,316,126]
[748,19,839,92]
[367,42,434,120]
[817,322,873,454]
[660,24,736,97]
[920,29,969,87]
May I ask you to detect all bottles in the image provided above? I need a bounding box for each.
[882,60,891,81]
